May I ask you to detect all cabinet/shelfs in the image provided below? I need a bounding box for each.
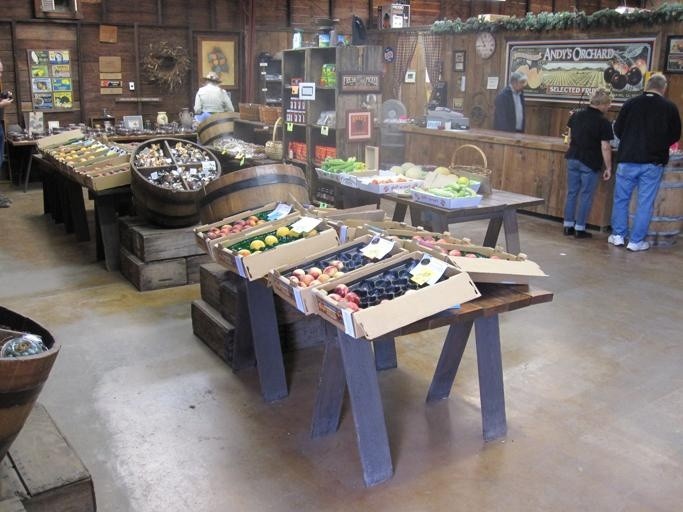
[256,55,282,108]
[279,43,386,212]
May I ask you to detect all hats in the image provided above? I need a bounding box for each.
[202,71,223,84]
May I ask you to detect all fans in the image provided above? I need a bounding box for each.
[379,98,407,130]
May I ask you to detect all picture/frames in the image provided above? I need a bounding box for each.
[195,33,240,93]
[337,70,384,94]
[451,48,466,73]
[344,108,375,143]
[297,82,316,101]
[662,34,682,74]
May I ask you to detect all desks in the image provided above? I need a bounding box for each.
[1,101,283,272]
[229,253,555,490]
[317,171,547,262]
[0,400,99,512]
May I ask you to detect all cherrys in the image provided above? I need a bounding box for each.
[603,59,647,90]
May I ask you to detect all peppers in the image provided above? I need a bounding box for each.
[320,157,356,172]
[428,182,476,198]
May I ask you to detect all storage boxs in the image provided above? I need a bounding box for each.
[313,165,485,211]
[187,189,551,343]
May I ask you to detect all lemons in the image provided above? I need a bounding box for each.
[237,249,251,257]
[276,226,317,238]
[264,235,278,246]
[250,240,265,251]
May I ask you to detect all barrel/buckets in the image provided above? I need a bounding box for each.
[128,137,221,227]
[0,303,62,461]
[196,112,239,146]
[193,164,311,224]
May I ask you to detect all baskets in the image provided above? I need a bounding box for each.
[264,117,294,161]
[448,144,493,196]
[258,105,282,126]
[238,102,261,121]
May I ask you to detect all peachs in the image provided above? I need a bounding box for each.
[413,235,476,258]
[207,215,266,240]
[290,261,360,313]
[490,255,501,259]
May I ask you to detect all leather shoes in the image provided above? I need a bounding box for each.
[574,230,592,240]
[563,226,575,235]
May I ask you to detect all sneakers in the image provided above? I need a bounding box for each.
[607,234,625,246]
[0,194,13,207]
[626,240,650,252]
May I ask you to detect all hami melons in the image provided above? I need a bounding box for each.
[433,166,450,177]
[390,162,422,179]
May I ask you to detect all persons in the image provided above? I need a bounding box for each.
[492,71,529,134]
[191,71,236,115]
[562,87,614,239]
[0,60,14,210]
[604,71,682,253]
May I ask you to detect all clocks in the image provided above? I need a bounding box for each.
[474,30,496,61]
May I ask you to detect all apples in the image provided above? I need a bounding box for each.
[458,176,468,184]
